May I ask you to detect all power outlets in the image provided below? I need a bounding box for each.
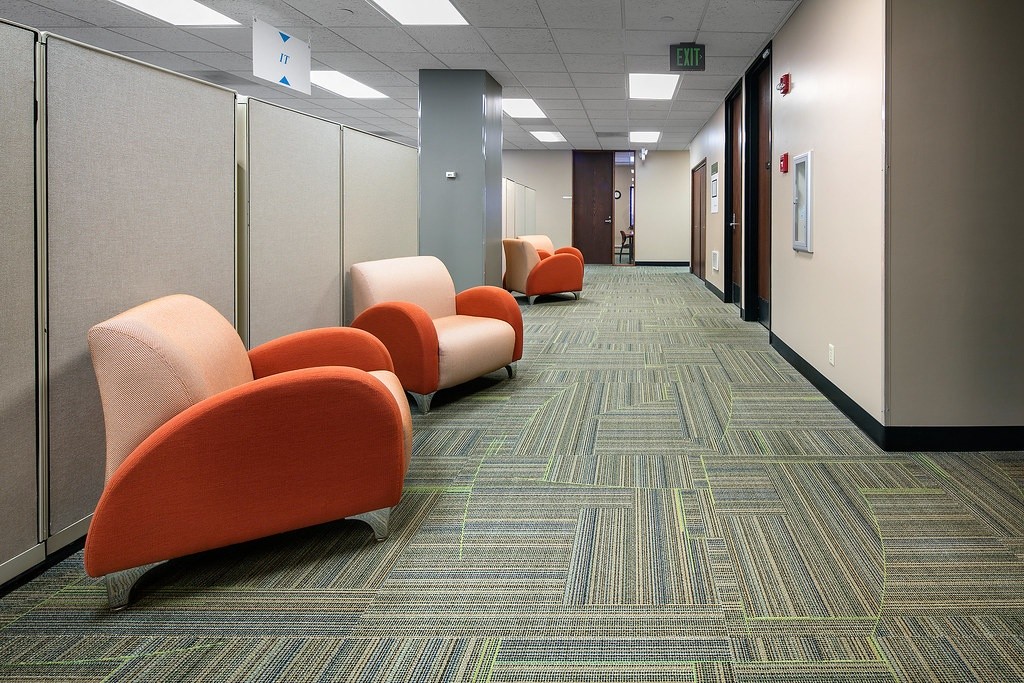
[828,344,835,367]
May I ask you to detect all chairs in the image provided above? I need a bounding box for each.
[619,230,633,264]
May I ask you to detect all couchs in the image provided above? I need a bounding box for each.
[84,293,412,613]
[502,238,583,306]
[516,235,585,278]
[349,255,523,415]
